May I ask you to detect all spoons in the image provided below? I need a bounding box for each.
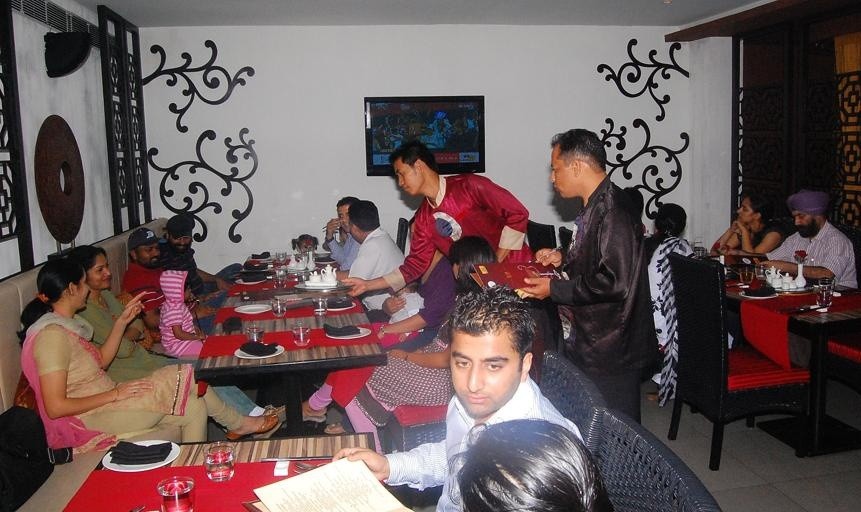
[294,461,331,470]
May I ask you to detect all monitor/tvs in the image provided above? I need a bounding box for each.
[365,96,485,176]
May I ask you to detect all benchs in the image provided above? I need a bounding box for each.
[1,216,176,512]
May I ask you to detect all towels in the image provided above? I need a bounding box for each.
[244,263,269,270]
[110,438,173,465]
[240,269,266,282]
[240,339,279,358]
[252,252,271,259]
[322,321,362,337]
[316,257,336,262]
[312,298,353,308]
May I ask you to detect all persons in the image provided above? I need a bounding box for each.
[68,248,290,440]
[157,215,243,307]
[754,189,860,369]
[642,200,736,407]
[342,136,557,354]
[623,185,651,238]
[512,130,664,426]
[370,109,481,153]
[17,254,281,454]
[122,229,230,336]
[709,190,791,256]
[157,269,209,359]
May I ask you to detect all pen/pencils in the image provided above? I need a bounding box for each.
[529,245,563,264]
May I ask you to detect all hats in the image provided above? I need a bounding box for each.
[166,215,194,240]
[126,226,161,250]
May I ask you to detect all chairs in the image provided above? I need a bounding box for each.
[387,214,861,511]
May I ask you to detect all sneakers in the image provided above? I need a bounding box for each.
[224,402,346,441]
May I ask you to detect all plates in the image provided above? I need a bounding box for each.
[327,326,371,340]
[234,345,285,361]
[328,303,359,311]
[103,438,181,472]
[234,304,273,315]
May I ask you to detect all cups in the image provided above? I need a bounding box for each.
[312,296,328,315]
[292,325,312,348]
[158,477,198,511]
[249,327,264,344]
[238,248,343,289]
[691,237,834,307]
[202,441,236,482]
[274,298,288,319]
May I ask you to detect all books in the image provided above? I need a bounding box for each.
[243,454,415,510]
[470,262,561,292]
